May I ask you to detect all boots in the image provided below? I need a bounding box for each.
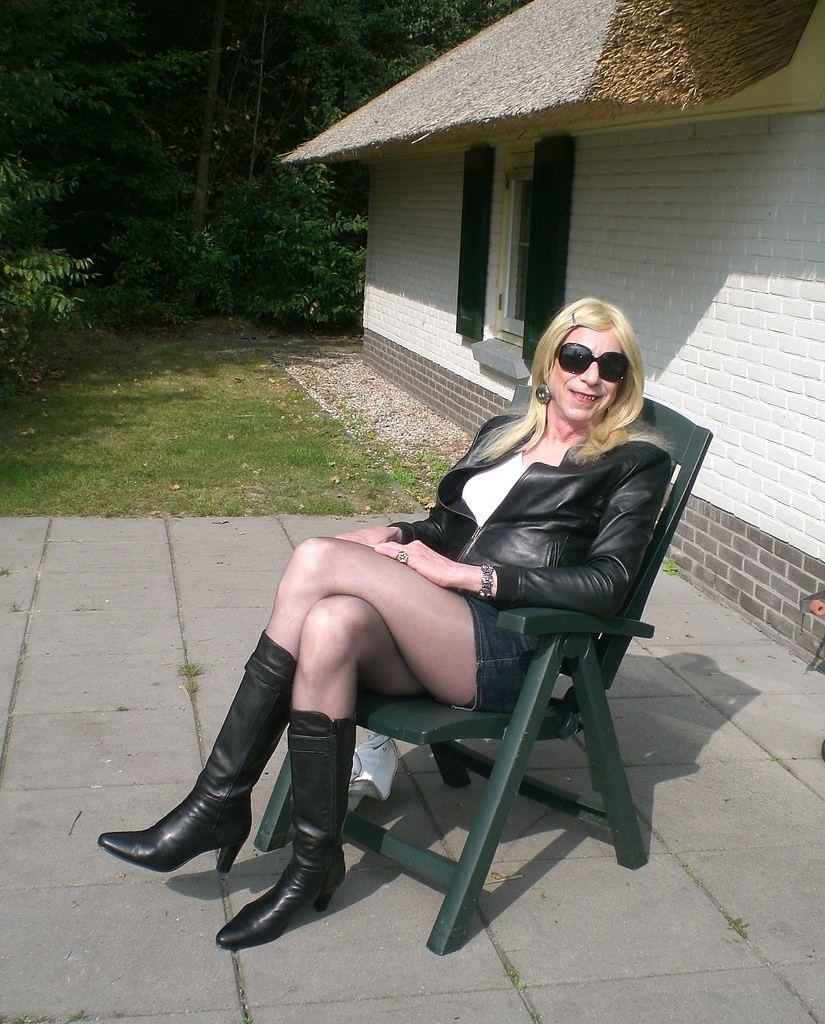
[96,627,298,873]
[213,707,356,949]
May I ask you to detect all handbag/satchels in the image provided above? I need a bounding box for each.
[342,733,399,814]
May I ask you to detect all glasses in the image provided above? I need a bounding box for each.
[557,342,629,382]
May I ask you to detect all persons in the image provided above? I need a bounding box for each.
[96,297,676,954]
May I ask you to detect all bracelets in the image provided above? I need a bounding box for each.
[478,565,494,600]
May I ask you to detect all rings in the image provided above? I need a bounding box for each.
[396,551,408,563]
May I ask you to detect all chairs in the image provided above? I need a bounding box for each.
[253,385,713,956]
